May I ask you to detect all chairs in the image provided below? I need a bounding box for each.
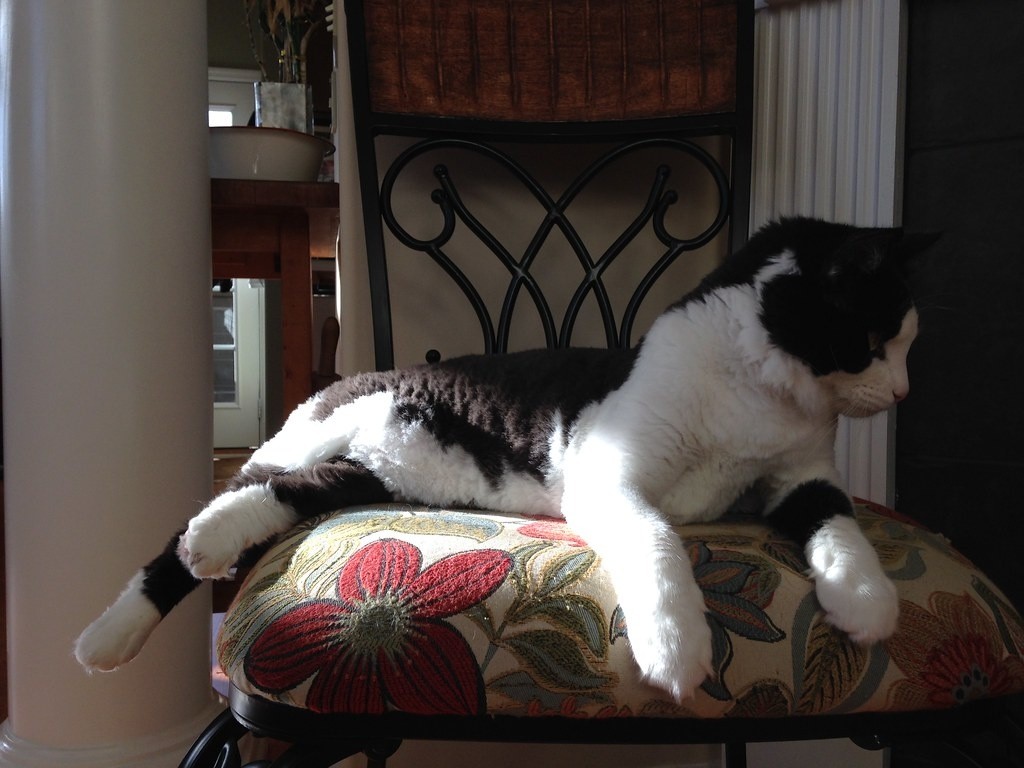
[184,0,1024,768]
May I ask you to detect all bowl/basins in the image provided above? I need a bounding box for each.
[208,126,336,182]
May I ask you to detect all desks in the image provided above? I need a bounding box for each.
[207,170,340,425]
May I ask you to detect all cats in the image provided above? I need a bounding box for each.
[74,216,948,704]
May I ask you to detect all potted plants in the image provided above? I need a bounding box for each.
[244,0,326,136]
